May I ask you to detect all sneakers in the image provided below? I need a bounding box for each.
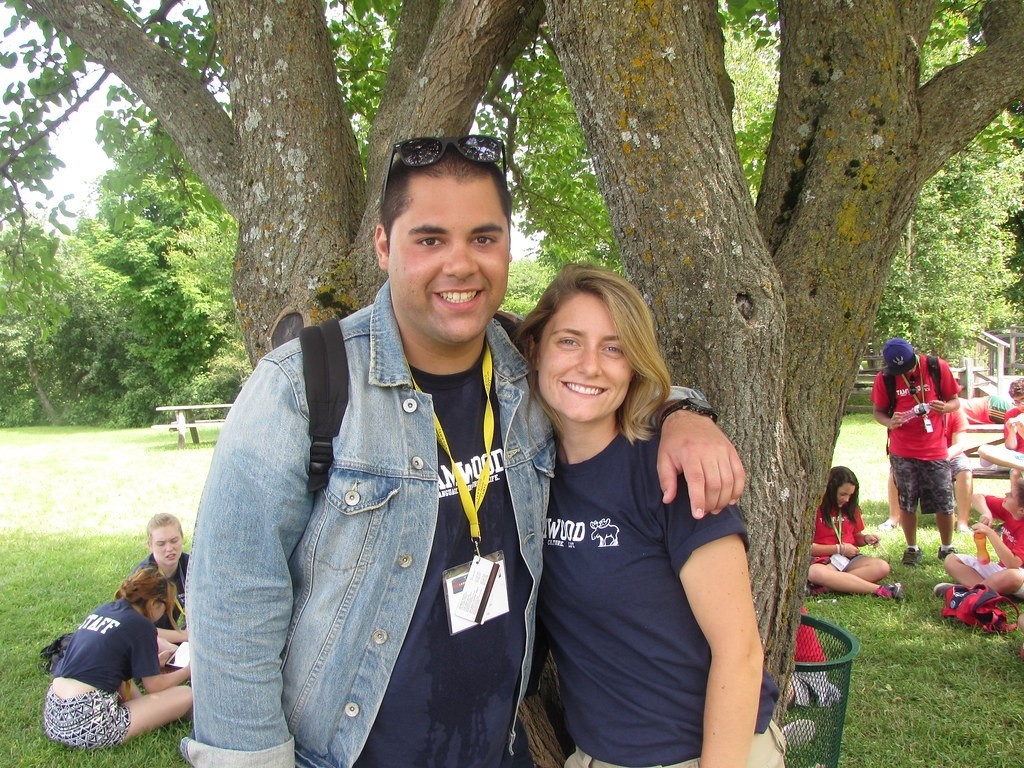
[956,523,974,535]
[874,518,899,531]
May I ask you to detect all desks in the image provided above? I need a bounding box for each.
[155,403,233,450]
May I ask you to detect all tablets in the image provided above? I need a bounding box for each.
[164,641,191,668]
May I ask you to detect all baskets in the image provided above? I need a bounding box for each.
[780,614,860,768]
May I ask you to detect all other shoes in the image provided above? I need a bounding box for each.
[937,547,958,564]
[933,582,956,596]
[883,581,901,598]
[902,547,922,564]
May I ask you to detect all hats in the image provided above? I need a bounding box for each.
[883,338,916,375]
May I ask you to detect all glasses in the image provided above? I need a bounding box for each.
[382,134,506,201]
[1011,398,1024,406]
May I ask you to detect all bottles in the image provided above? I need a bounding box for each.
[900,403,931,422]
[973,531,991,565]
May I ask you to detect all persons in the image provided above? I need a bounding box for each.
[40,135,1024,768]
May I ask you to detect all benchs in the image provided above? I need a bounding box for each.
[151,422,225,429]
[967,454,1011,480]
[171,419,226,424]
[967,422,1005,433]
